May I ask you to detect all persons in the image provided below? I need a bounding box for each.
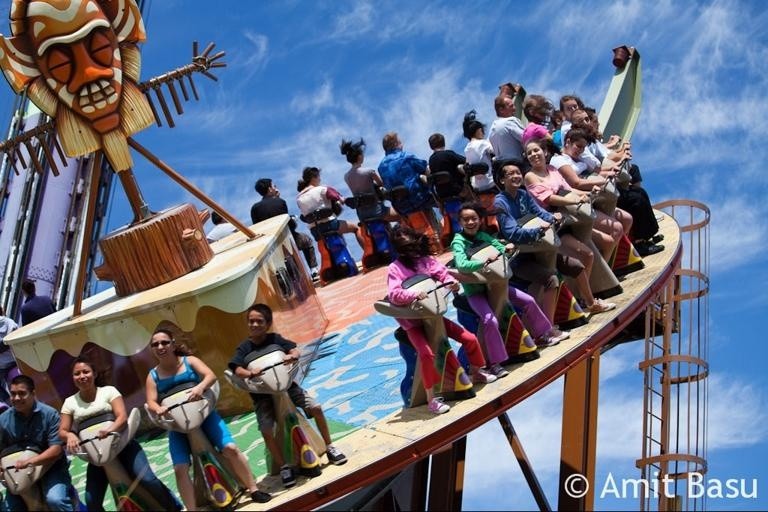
[57,355,185,511]
[206,209,240,244]
[144,327,272,512]
[295,166,367,251]
[226,302,348,488]
[337,96,667,415]
[249,177,320,278]
[0,280,58,405]
[0,375,77,512]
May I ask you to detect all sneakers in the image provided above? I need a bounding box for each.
[427,397,451,413]
[536,327,571,347]
[586,298,616,315]
[468,369,498,382]
[489,364,509,377]
[326,447,347,465]
[248,490,271,502]
[634,233,665,256]
[279,465,298,487]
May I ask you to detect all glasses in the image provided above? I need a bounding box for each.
[150,340,173,348]
